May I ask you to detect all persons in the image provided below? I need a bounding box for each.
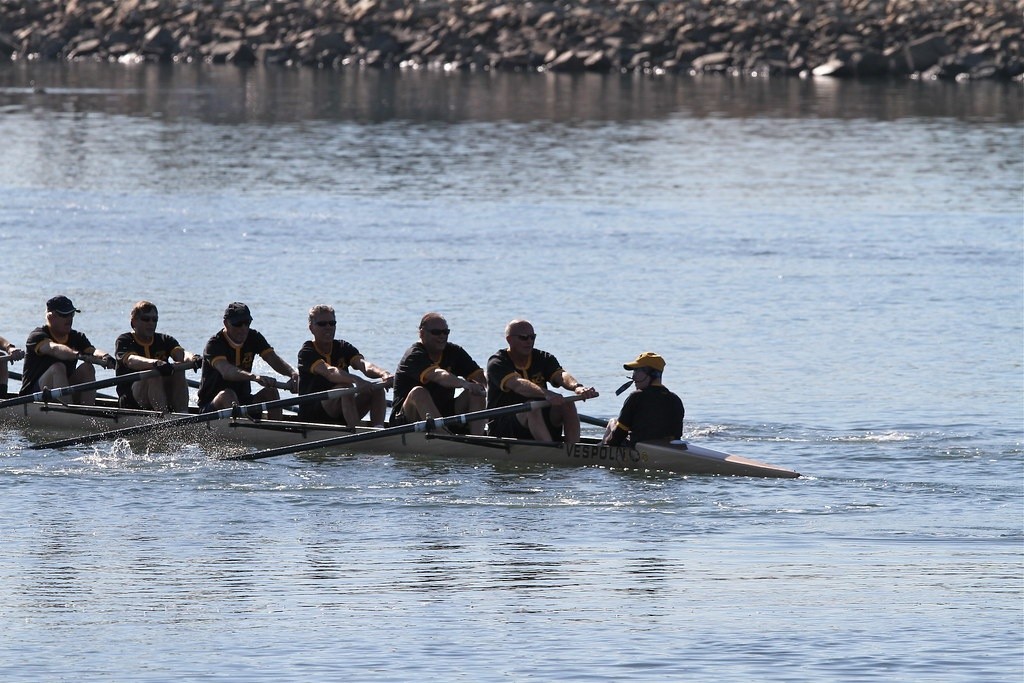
[20,295,116,406]
[198,302,298,421]
[389,312,487,435]
[298,304,394,428]
[598,352,685,446]
[0,336,25,398]
[114,301,202,414]
[486,320,595,443]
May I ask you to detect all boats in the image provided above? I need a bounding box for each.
[0,36,1024,86]
[0,390,803,482]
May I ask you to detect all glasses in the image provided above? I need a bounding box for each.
[508,334,536,341]
[58,312,75,317]
[137,315,158,322]
[229,322,251,327]
[423,328,450,336]
[312,320,336,326]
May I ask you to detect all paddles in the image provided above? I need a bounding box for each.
[220,391,600,460]
[0,356,192,409]
[26,383,357,451]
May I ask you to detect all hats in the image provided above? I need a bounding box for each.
[623,352,666,371]
[47,295,81,315]
[224,302,253,322]
